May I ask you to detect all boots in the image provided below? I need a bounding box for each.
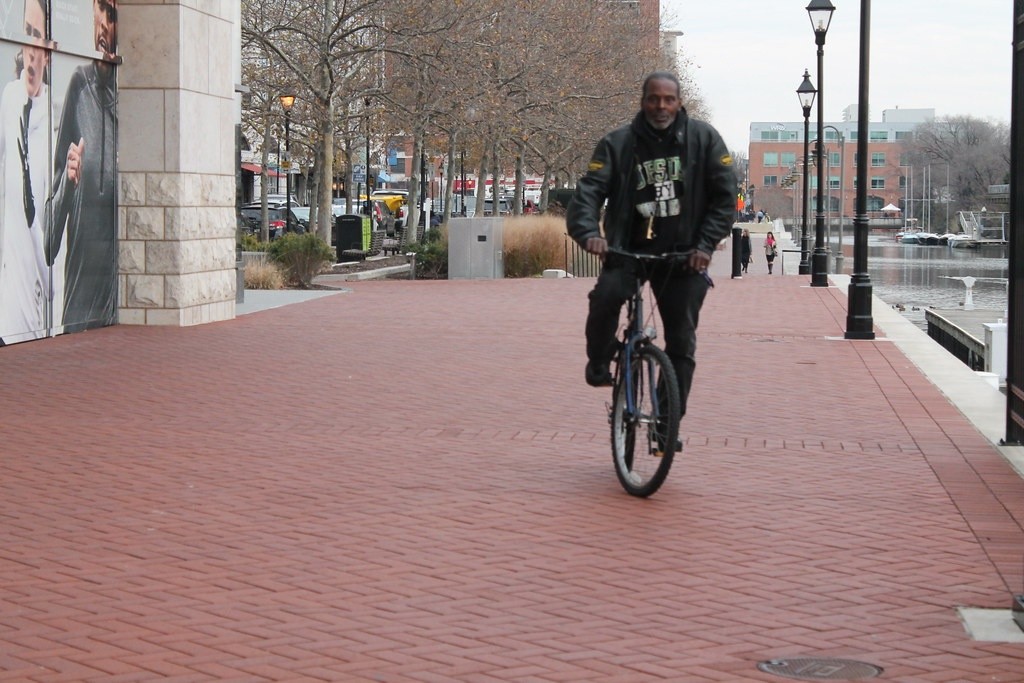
[768,264,773,274]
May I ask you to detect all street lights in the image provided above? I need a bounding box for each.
[780,126,845,274]
[797,69,817,275]
[280,86,296,234]
[806,0,836,285]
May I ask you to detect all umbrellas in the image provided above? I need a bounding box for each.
[880,203,901,211]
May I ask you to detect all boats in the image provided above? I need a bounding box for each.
[947,234,977,247]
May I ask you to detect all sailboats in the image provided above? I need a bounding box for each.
[896,162,956,245]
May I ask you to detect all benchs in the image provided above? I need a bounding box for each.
[342,230,386,262]
[382,225,425,255]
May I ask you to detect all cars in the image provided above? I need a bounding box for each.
[331,191,421,230]
[431,185,553,219]
[240,194,319,247]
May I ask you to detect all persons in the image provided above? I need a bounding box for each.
[741,228,752,273]
[430,210,441,226]
[757,209,765,223]
[739,209,745,222]
[43,0,120,332]
[748,211,756,220]
[564,72,738,452]
[763,232,777,274]
[0,0,55,345]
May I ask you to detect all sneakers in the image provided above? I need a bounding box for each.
[583,352,610,384]
[649,415,684,451]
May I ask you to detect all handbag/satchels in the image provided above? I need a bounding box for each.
[749,255,754,263]
[773,250,778,256]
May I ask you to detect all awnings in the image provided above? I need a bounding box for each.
[241,162,287,177]
[377,172,398,183]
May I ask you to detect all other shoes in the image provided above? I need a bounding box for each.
[742,267,748,273]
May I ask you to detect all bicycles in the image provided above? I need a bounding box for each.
[590,249,695,500]
